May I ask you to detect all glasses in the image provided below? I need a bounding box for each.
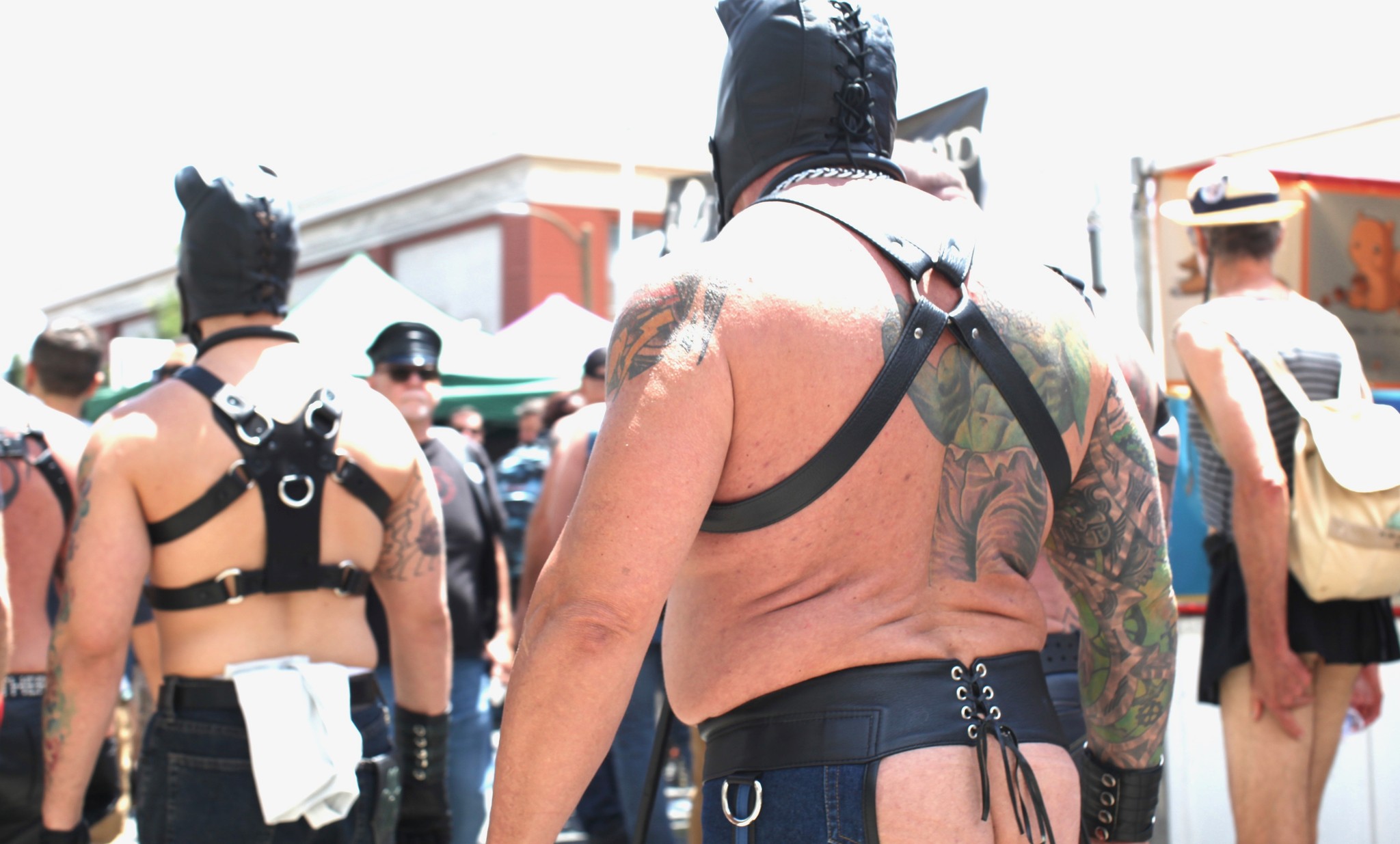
[456,426,479,435]
[387,364,438,384]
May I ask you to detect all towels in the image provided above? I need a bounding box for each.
[230,655,365,831]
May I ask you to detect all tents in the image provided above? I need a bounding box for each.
[0,253,617,455]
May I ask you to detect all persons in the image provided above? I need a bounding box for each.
[363,321,512,844]
[17,328,152,839]
[457,351,703,844]
[0,376,159,844]
[43,164,452,844]
[490,0,1177,844]
[1162,157,1400,844]
[882,139,1163,746]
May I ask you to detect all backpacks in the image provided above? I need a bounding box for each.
[1204,297,1400,601]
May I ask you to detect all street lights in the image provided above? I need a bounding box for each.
[494,199,592,315]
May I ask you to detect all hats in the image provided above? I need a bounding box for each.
[367,321,443,365]
[1160,160,1305,227]
